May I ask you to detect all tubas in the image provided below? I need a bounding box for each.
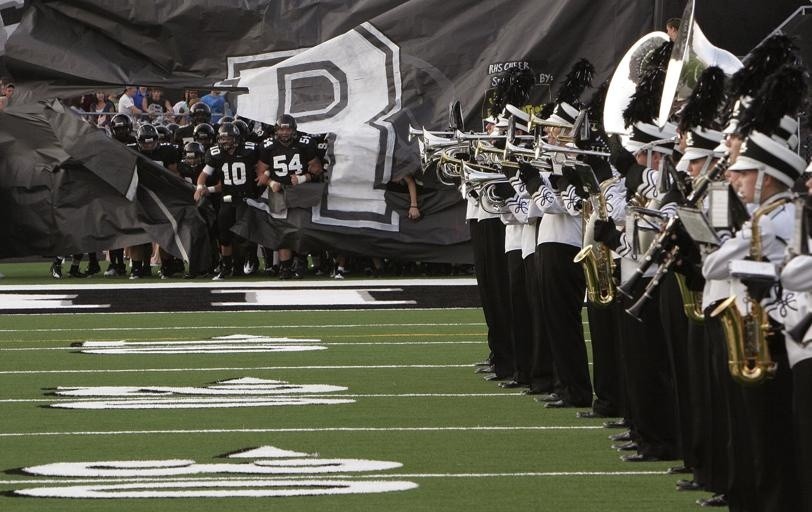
[602,0,749,151]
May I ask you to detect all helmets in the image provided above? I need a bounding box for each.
[218,122,240,149]
[216,115,236,127]
[136,124,159,153]
[192,123,215,146]
[109,113,132,140]
[156,126,171,146]
[230,120,249,141]
[189,102,211,125]
[276,115,296,142]
[184,142,205,168]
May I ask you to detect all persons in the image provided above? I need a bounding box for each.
[1,83,475,283]
[457,16,812,511]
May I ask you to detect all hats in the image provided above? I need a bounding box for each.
[495,103,530,133]
[680,125,723,162]
[728,130,807,189]
[721,94,799,147]
[546,101,580,127]
[482,109,497,125]
[625,120,677,156]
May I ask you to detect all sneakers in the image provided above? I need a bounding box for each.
[244,256,256,275]
[84,265,100,277]
[184,271,214,281]
[360,264,436,277]
[263,264,345,280]
[68,268,89,279]
[212,267,233,280]
[104,264,126,276]
[157,267,169,280]
[128,267,151,280]
[50,264,63,280]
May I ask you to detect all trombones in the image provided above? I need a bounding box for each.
[408,108,610,213]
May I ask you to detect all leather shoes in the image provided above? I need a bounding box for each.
[669,466,695,474]
[577,410,607,419]
[498,380,522,389]
[483,375,512,382]
[609,428,632,441]
[697,494,728,507]
[474,359,496,373]
[521,387,553,395]
[621,454,658,462]
[611,443,640,451]
[677,479,708,491]
[534,393,577,408]
[602,421,627,428]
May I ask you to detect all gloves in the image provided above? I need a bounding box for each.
[563,165,583,188]
[594,216,616,248]
[606,133,636,176]
[517,158,540,184]
[495,182,514,199]
[583,154,612,181]
[742,255,776,303]
[671,170,692,197]
[659,249,693,275]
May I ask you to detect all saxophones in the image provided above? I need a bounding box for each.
[710,195,804,382]
[573,181,620,304]
